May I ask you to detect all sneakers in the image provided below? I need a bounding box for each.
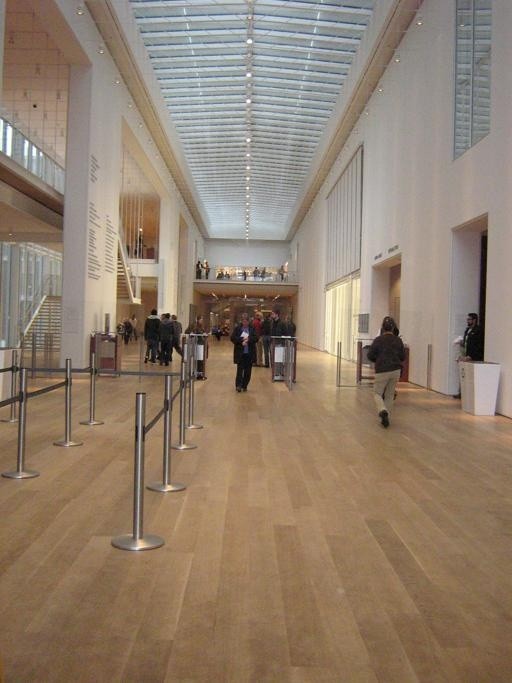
[379,411,389,427]
[144,356,172,366]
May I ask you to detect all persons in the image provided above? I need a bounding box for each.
[367,318,405,428]
[134,227,146,258]
[231,317,259,392]
[250,310,296,368]
[195,261,286,282]
[380,316,399,399]
[117,309,231,380]
[453,313,484,399]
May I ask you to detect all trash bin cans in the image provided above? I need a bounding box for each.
[459,360,502,416]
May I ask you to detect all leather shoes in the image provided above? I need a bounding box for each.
[238,389,247,392]
